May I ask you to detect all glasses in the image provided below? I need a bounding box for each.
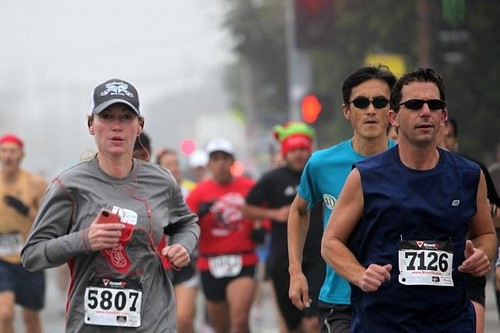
[349,96,391,109]
[393,99,446,111]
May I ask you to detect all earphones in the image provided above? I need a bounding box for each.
[90,123,94,135]
[138,124,142,137]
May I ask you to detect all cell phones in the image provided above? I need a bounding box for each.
[97,208,121,224]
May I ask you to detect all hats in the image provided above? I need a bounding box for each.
[273,122,315,156]
[0,133,23,147]
[207,138,237,161]
[89,78,143,117]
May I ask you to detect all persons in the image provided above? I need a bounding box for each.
[21,79,199,333]
[133,122,328,333]
[321,68,497,333]
[288,66,398,333]
[0,133,46,333]
[386,116,500,333]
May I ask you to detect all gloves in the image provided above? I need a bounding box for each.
[250,227,265,244]
[197,201,214,217]
